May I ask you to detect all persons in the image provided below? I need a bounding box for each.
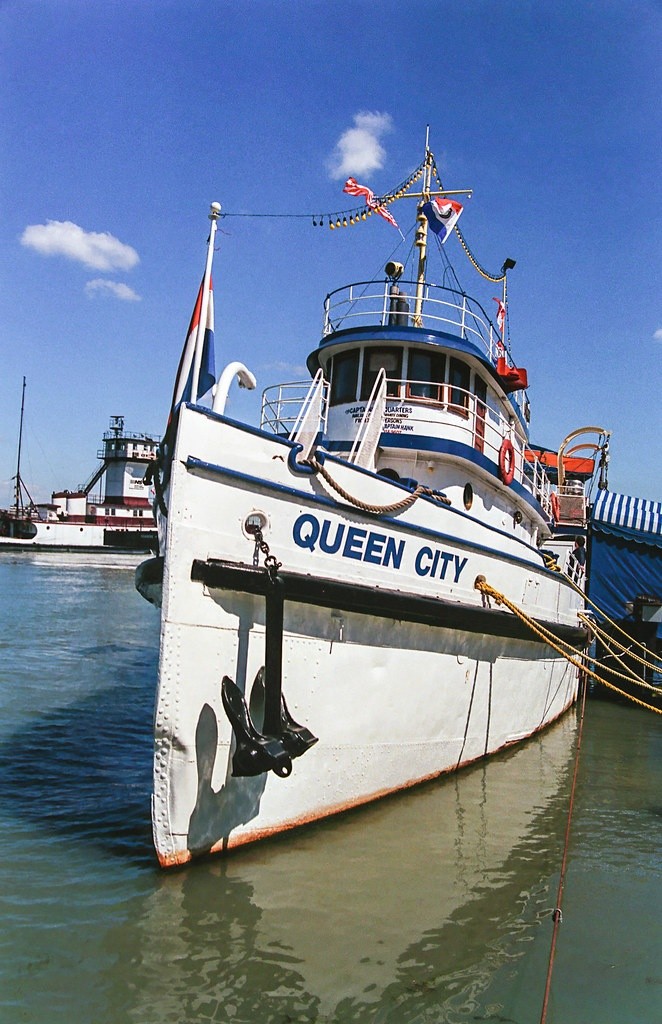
[568,536,586,584]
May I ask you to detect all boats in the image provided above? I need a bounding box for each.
[1,376,165,549]
[131,122,615,863]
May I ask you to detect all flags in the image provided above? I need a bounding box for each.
[420,193,464,244]
[492,297,506,334]
[342,176,399,228]
[159,269,216,472]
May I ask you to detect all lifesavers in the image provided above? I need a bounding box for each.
[499,439,515,486]
[551,490,560,522]
